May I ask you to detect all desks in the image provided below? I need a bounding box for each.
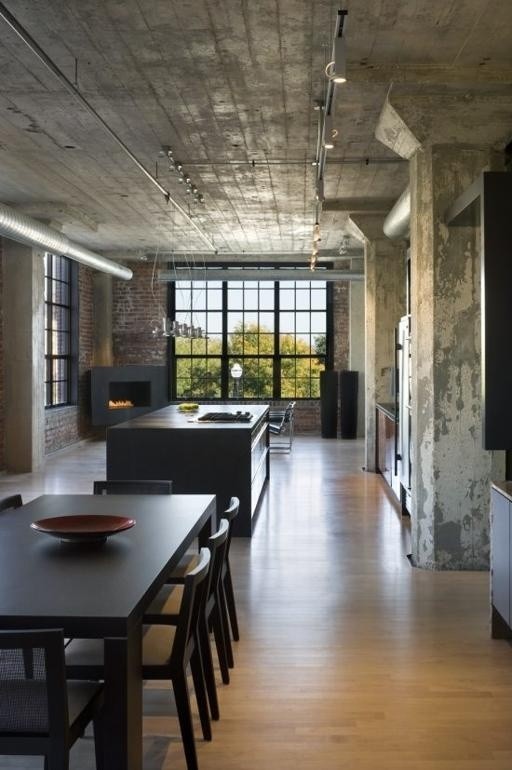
[1,493,217,769]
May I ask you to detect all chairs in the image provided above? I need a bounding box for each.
[269,401,297,454]
[59,547,215,770]
[2,626,103,769]
[1,493,24,512]
[141,517,232,724]
[164,493,238,671]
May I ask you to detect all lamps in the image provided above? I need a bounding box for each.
[147,146,207,339]
[309,9,349,272]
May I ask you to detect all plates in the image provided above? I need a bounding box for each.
[30,514,135,542]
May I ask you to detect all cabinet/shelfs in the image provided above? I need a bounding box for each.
[488,480,512,639]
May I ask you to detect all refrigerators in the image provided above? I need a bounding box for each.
[393,315,412,517]
